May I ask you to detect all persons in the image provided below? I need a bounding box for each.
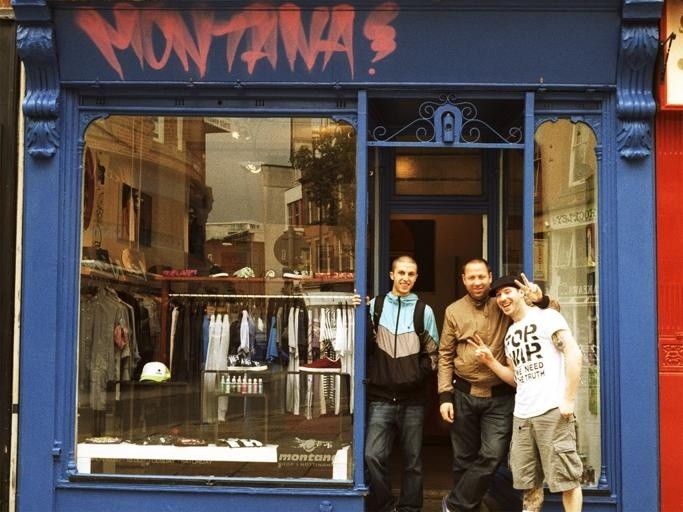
[466,275,584,512]
[352,255,440,512]
[437,258,560,512]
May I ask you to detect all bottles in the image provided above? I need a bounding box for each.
[598,466,608,490]
[219,375,263,394]
[64,452,78,475]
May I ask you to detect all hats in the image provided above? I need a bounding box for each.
[488,275,525,298]
[139,361,172,384]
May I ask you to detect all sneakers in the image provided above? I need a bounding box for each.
[226,354,268,372]
[298,356,342,375]
[442,493,454,512]
[209,264,229,278]
[283,267,313,280]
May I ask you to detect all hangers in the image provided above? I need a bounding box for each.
[201,295,353,326]
[81,273,202,314]
